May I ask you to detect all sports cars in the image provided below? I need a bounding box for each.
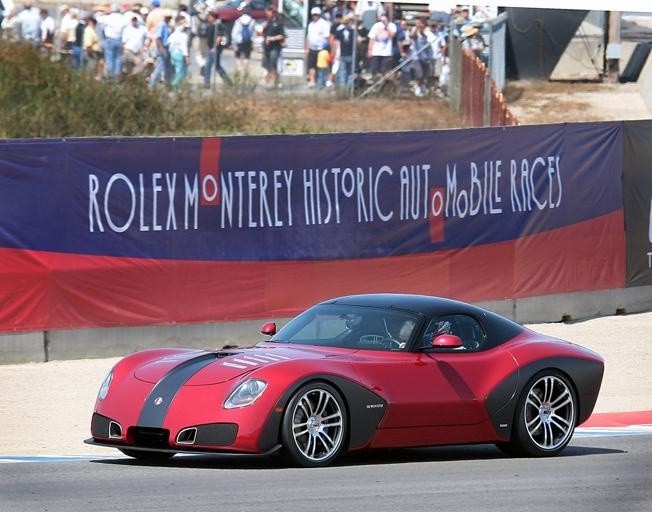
[82,293,604,465]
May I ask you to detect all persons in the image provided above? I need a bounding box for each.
[399,320,464,349]
[1,1,493,99]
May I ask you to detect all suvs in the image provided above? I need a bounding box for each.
[204,1,300,48]
